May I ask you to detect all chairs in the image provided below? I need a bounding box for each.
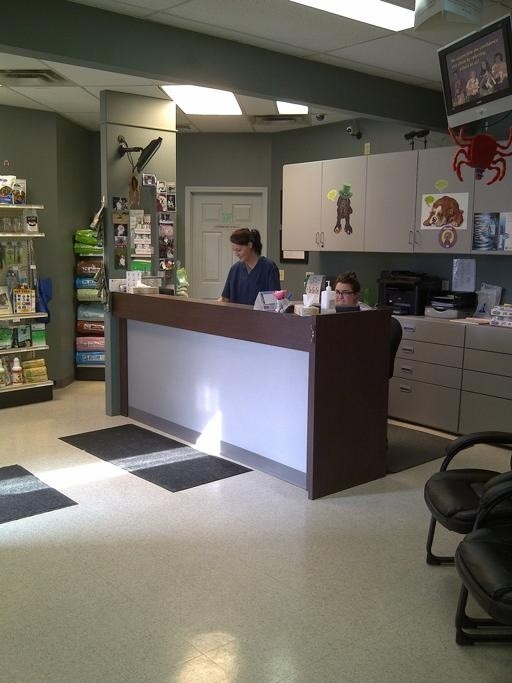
[424,428,511,646]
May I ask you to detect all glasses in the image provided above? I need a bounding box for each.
[335,290,355,297]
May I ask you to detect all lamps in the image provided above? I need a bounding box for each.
[118,133,162,173]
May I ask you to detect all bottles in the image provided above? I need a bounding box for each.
[11,356,24,385]
[0,358,7,387]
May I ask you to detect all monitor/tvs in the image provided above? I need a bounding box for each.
[136,137,162,172]
[437,13,512,129]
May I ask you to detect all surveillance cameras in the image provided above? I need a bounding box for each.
[404,131,416,140]
[316,114,324,121]
[416,130,430,138]
[346,126,354,134]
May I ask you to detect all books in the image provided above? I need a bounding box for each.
[449,316,491,325]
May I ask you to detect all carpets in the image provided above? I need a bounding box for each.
[0,464,78,525]
[387,423,454,474]
[59,423,253,493]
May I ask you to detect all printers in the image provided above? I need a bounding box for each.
[378,269,441,316]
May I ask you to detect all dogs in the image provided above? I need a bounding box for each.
[423,195,464,228]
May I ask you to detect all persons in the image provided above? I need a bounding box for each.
[492,52,507,90]
[217,226,282,306]
[452,69,467,105]
[465,70,479,100]
[480,59,494,96]
[332,270,372,309]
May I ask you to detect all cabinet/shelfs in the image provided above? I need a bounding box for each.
[0,204,54,409]
[364,146,473,255]
[283,156,364,251]
[476,140,510,255]
[388,314,512,444]
